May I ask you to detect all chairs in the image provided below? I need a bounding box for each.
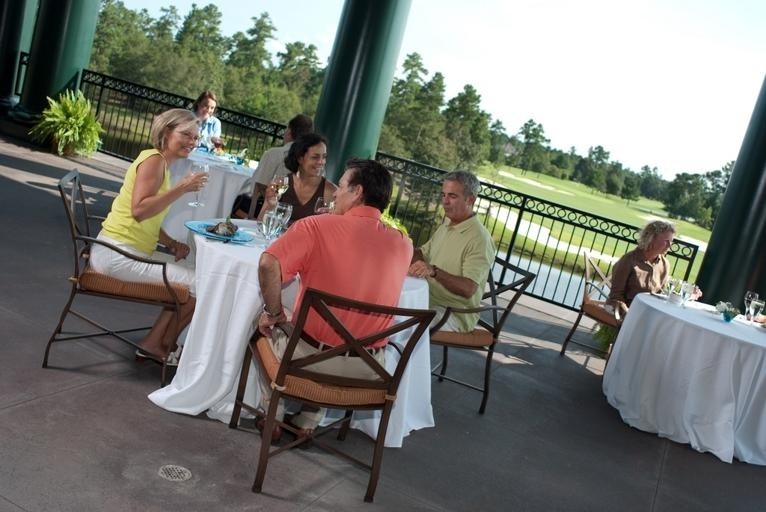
[225,284,438,505]
[431,251,536,417]
[556,249,633,360]
[40,169,189,391]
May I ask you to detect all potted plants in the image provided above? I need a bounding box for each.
[26,87,106,159]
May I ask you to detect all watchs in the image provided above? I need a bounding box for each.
[263,304,286,318]
[430,265,438,278]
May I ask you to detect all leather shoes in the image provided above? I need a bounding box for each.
[255,414,282,444]
[283,411,315,444]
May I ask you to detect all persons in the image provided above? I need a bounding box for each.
[409,171,496,334]
[255,157,414,444]
[257,133,340,227]
[187,90,222,147]
[231,114,314,218]
[605,221,676,325]
[89,108,212,367]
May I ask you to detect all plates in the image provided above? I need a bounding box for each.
[184,220,255,244]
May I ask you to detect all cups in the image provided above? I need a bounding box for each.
[262,212,279,240]
[275,204,293,231]
[188,164,209,208]
[271,176,289,204]
[750,299,765,324]
[314,197,331,214]
[744,290,759,320]
[206,137,215,153]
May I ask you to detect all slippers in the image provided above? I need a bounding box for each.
[131,348,180,367]
[165,348,185,361]
